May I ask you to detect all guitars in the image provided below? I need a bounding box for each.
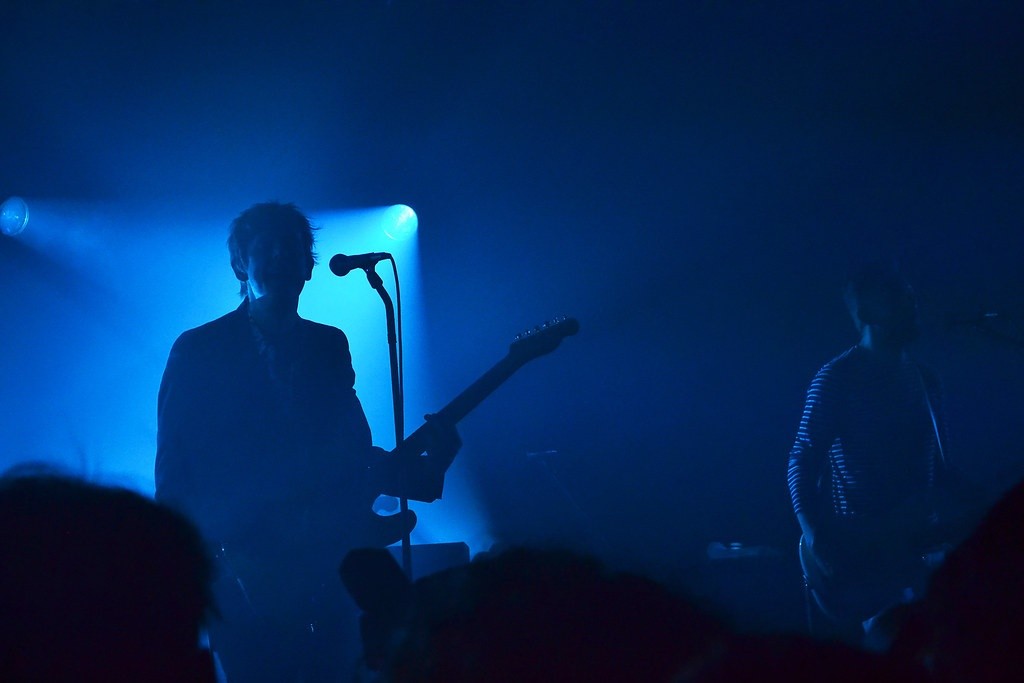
[797,471,1024,637]
[205,313,586,683]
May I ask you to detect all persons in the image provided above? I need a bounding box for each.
[788,265,950,646]
[0,466,1024,683]
[151,203,462,683]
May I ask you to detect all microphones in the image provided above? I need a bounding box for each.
[330,252,391,277]
